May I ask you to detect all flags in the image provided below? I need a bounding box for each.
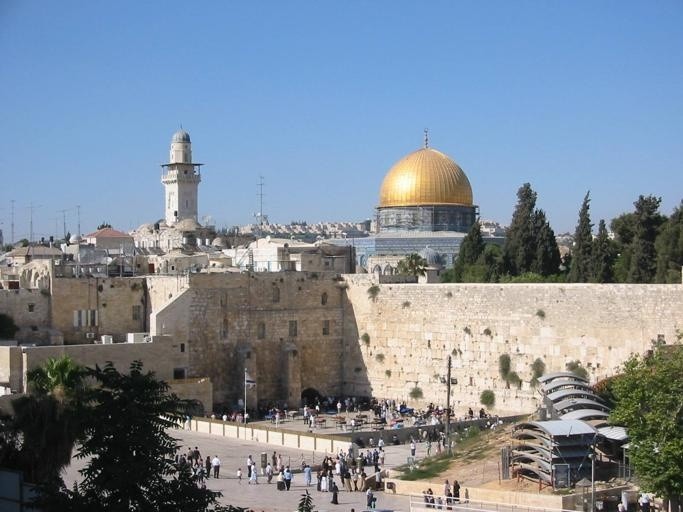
[244,372,256,390]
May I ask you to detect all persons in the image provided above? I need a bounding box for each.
[617,500,624,512]
[144,334,148,342]
[639,494,643,510]
[641,490,650,512]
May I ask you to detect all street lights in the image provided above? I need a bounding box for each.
[439,355,458,440]
